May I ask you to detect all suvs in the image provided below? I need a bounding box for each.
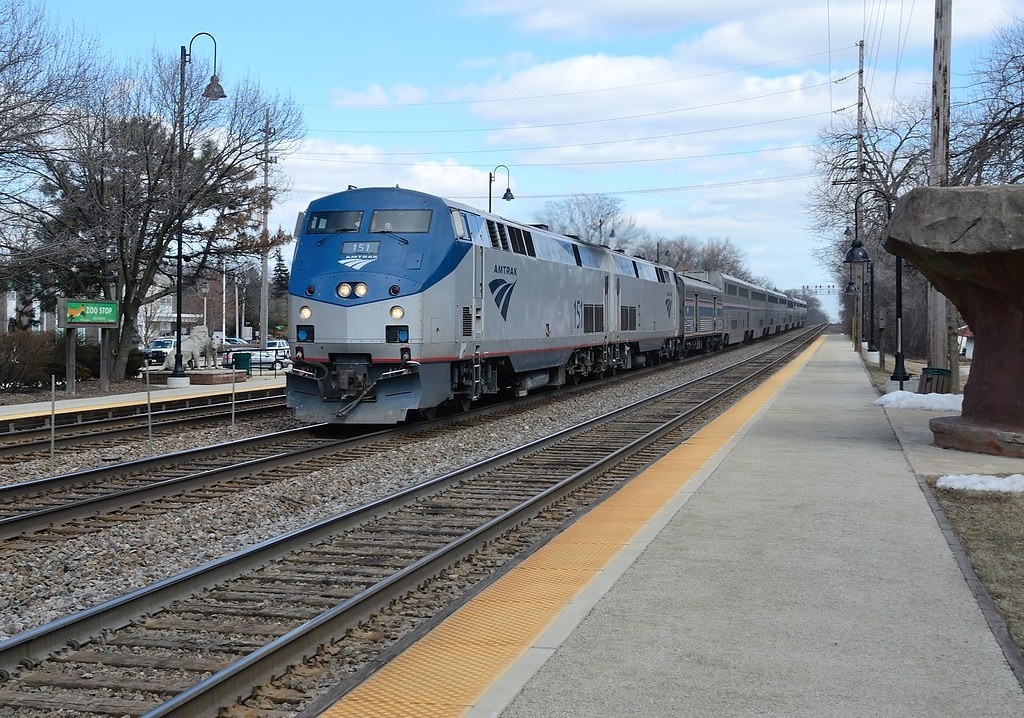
[142,338,183,367]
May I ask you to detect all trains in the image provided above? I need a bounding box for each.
[283,184,809,426]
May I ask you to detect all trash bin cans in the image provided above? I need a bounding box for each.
[917,368,952,394]
[234,353,251,377]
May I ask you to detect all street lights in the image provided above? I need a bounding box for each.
[598,214,616,246]
[200,283,211,326]
[166,32,228,387]
[657,238,670,265]
[845,260,880,364]
[844,188,918,392]
[488,164,515,214]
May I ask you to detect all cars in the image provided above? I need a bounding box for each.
[216,336,291,371]
